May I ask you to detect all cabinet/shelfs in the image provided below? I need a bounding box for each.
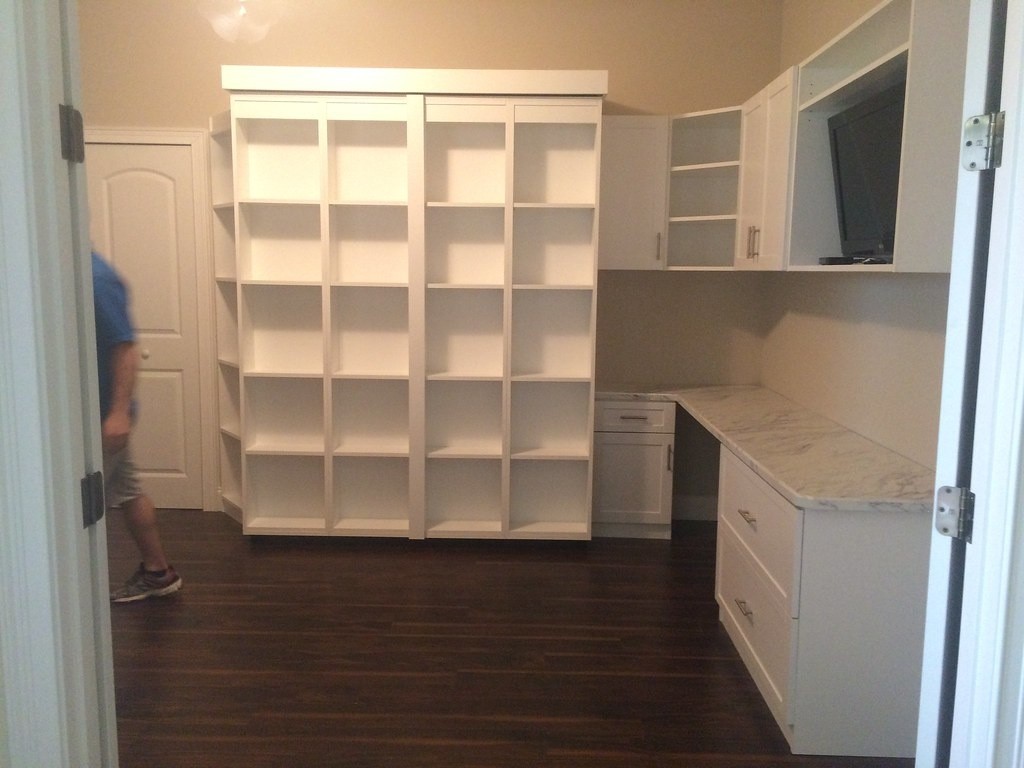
[598,114,669,270]
[714,442,933,758]
[666,106,742,270]
[786,0,970,272]
[734,64,799,272]
[593,399,676,539]
[208,65,610,540]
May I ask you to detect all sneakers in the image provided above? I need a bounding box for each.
[110,561,182,602]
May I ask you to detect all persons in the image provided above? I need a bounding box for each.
[92,252,181,603]
